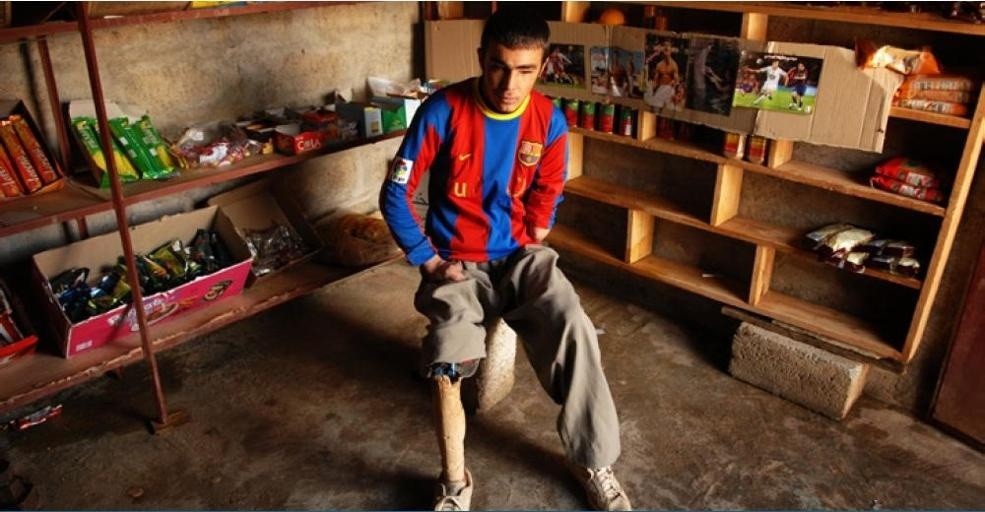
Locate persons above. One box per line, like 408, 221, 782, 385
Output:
536, 34, 813, 111
380, 9, 637, 512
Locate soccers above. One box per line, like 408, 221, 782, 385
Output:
805, 104, 812, 113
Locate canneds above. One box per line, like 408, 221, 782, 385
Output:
552, 99, 634, 137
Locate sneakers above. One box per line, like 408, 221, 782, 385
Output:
434, 465, 474, 511
572, 463, 632, 511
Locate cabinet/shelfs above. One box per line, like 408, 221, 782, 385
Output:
534, 0, 984, 377
0, 2, 453, 410
